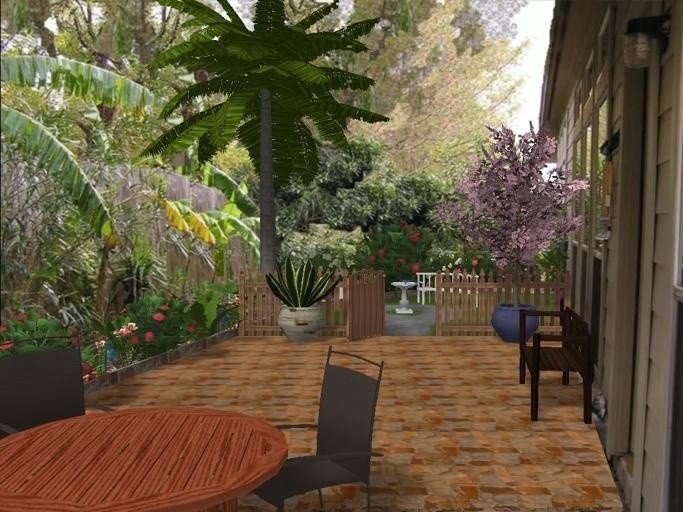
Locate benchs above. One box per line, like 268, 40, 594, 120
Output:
415, 271, 481, 308
518, 307, 597, 426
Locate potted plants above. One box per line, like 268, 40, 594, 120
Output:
265, 255, 343, 345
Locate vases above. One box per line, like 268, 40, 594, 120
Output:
492, 299, 539, 344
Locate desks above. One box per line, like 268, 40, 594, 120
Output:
1, 407, 287, 511
391, 282, 419, 314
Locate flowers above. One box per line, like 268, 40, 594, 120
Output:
439, 120, 593, 303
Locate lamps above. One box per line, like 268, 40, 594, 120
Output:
621, 13, 672, 69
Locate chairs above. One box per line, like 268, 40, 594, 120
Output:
254, 344, 385, 512
0, 346, 85, 438
